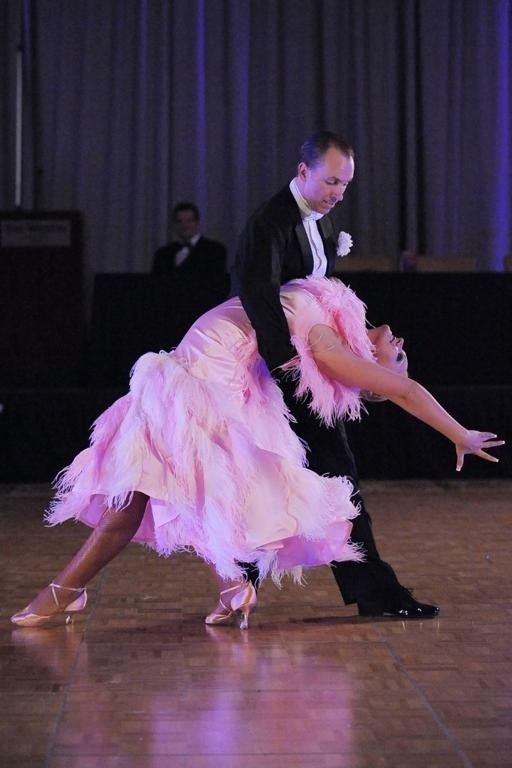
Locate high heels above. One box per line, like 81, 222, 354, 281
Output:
205, 581, 256, 629
10, 582, 87, 627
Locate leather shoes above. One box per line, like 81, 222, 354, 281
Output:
358, 585, 439, 619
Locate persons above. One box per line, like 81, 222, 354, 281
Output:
9, 272, 507, 632
398, 244, 426, 274
234, 132, 439, 625
145, 202, 228, 275
